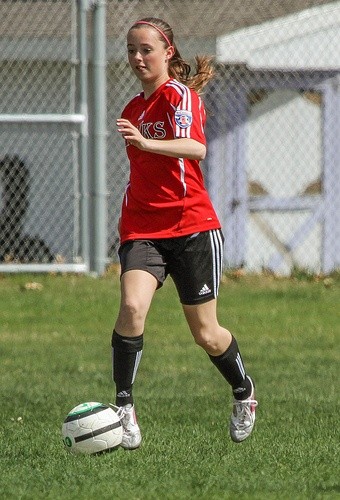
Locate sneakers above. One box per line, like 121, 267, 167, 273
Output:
230, 375, 258, 443
108, 402, 142, 449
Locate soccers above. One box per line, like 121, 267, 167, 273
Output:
61, 401, 124, 456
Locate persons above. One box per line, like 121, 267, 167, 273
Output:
112, 18, 259, 448
